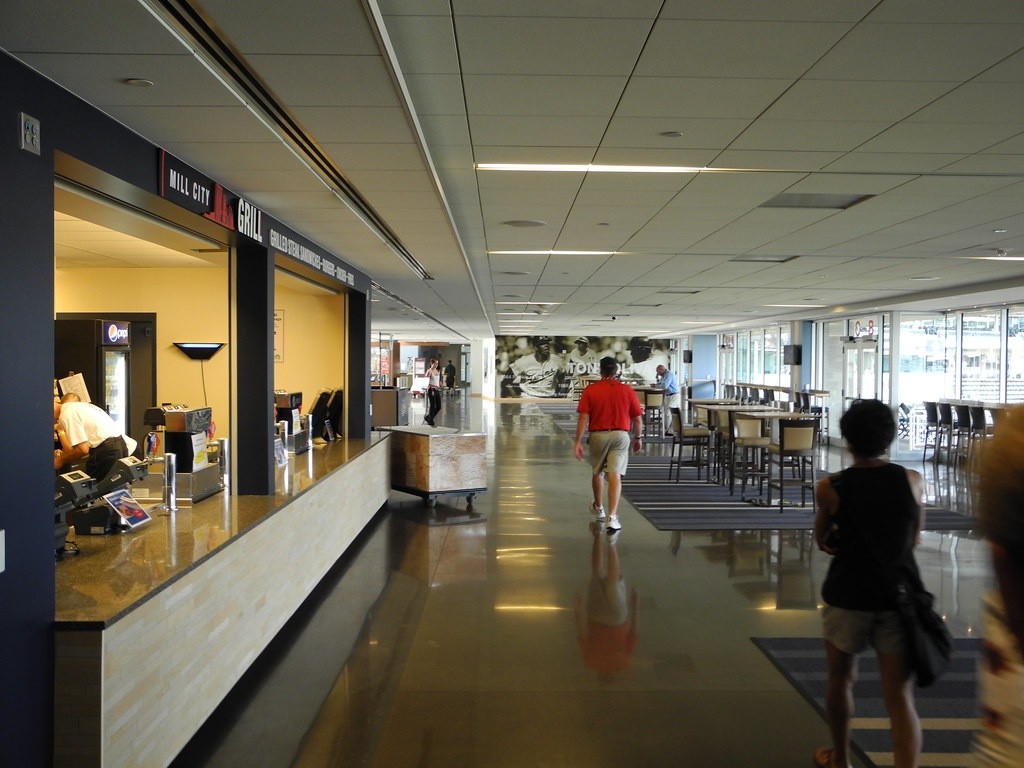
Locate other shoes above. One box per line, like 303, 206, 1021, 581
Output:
813, 745, 852, 768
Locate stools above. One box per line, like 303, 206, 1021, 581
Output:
569, 377, 830, 514
954, 404, 973, 474
922, 401, 941, 467
936, 402, 958, 468
969, 406, 994, 452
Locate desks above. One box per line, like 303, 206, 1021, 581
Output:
684, 398, 751, 469
694, 404, 784, 484
721, 382, 830, 444
934, 398, 1010, 421
632, 386, 665, 438
736, 412, 823, 507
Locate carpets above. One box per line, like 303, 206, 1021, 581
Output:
584, 455, 989, 531
552, 419, 715, 444
749, 636, 986, 768
536, 402, 581, 414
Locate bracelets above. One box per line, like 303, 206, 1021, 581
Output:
634, 437, 642, 439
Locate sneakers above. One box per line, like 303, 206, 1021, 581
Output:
605, 516, 621, 529
588, 498, 605, 518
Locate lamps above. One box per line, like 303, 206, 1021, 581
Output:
862, 335, 877, 342
172, 342, 226, 360
839, 336, 855, 342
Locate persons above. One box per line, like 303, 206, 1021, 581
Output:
649, 365, 679, 437
973, 405, 1024, 768
568, 337, 596, 398
425, 358, 444, 425
444, 360, 455, 389
813, 399, 924, 768
574, 357, 643, 530
621, 337, 669, 387
501, 336, 566, 398
54, 392, 129, 485
573, 523, 640, 682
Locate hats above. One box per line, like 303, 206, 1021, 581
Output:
626, 337, 652, 350
532, 336, 552, 343
573, 336, 589, 344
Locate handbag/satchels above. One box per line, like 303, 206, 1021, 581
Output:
901, 587, 955, 686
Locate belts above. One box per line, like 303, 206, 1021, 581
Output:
600, 428, 621, 431
667, 393, 674, 396
103, 435, 123, 445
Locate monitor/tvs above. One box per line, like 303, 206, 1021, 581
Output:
783, 345, 800, 365
684, 350, 692, 364
